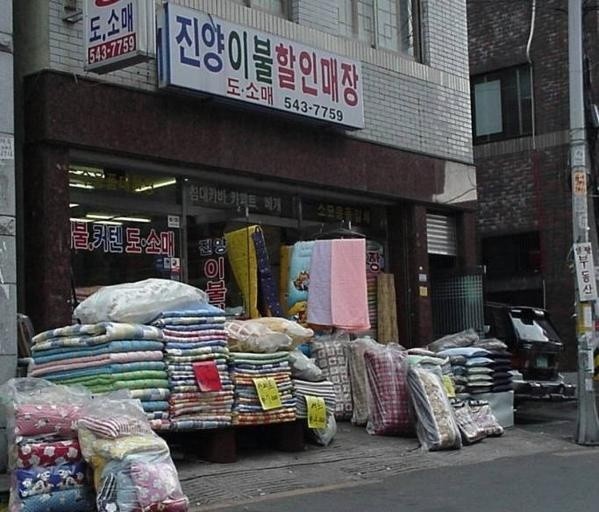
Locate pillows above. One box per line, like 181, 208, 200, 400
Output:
224, 317, 514, 454
5, 395, 189, 512
73, 278, 207, 328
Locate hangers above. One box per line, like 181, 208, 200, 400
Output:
307, 222, 344, 241
314, 219, 367, 241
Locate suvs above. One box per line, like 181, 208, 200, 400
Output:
481, 298, 578, 408
